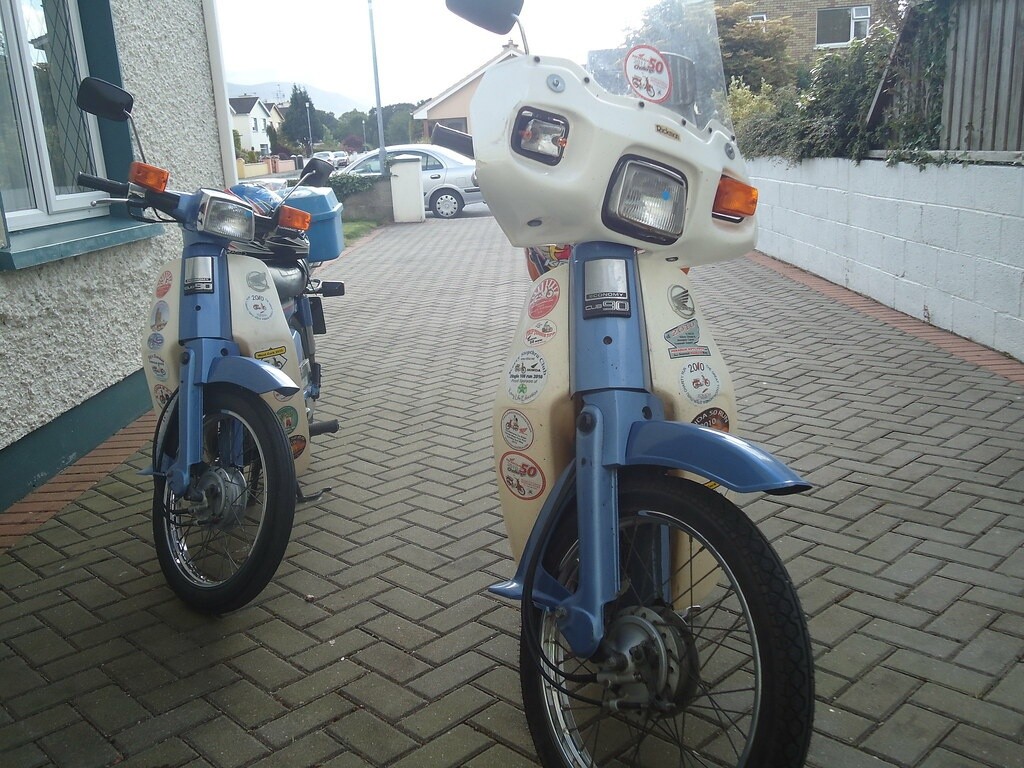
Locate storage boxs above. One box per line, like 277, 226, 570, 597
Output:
269, 187, 345, 261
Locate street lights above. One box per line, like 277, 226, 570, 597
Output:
363, 120, 367, 152
306, 102, 313, 153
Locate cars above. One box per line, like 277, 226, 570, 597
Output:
313, 151, 338, 171
333, 152, 350, 166
335, 142, 481, 218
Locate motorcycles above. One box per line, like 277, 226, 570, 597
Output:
72, 76, 346, 610
451, 1, 816, 768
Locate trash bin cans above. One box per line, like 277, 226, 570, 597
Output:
389, 154, 425, 223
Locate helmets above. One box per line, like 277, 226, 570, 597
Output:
225, 184, 285, 223
